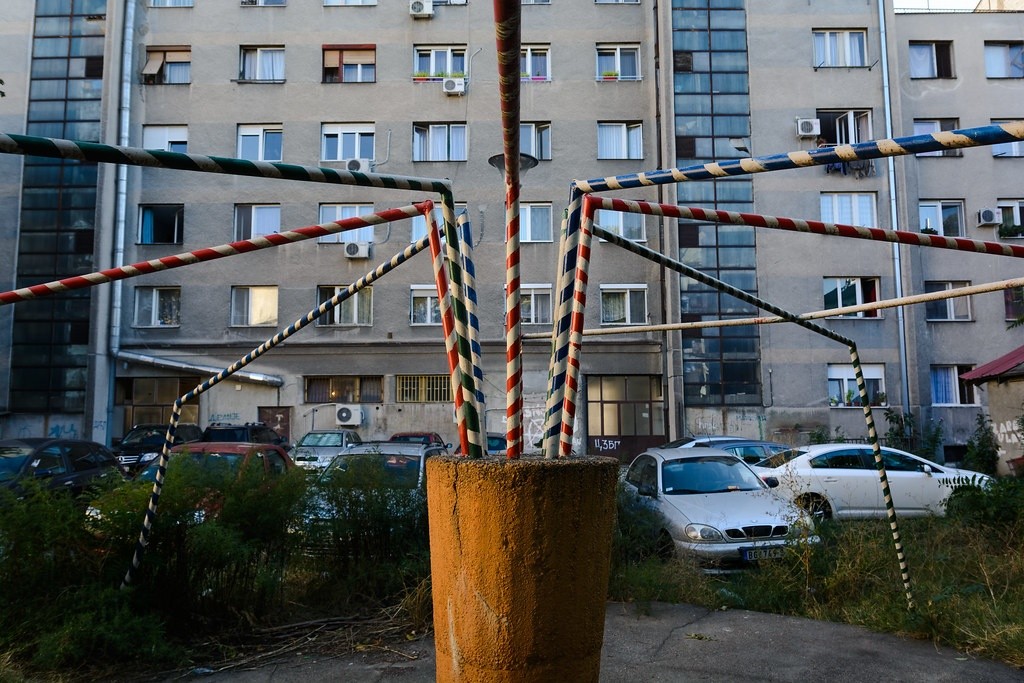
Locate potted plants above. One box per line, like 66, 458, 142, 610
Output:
829, 389, 887, 407
997, 223, 1024, 237
602, 72, 619, 82
520, 71, 546, 81
147, 76, 154, 84
414, 72, 464, 82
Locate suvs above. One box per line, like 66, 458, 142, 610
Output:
111, 423, 203, 476
201, 421, 295, 463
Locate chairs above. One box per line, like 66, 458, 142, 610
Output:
694, 465, 725, 493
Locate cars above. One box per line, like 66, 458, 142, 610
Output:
383, 432, 452, 468
617, 448, 820, 575
681, 439, 796, 489
453, 431, 508, 458
280, 428, 362, 483
74, 441, 306, 563
0, 437, 128, 503
641, 434, 790, 473
737, 443, 1003, 524
280, 441, 451, 565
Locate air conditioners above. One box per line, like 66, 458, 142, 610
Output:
335, 403, 361, 425
409, 0, 432, 16
442, 77, 464, 94
346, 158, 371, 173
796, 118, 820, 137
344, 242, 368, 257
441, 241, 462, 257
976, 208, 1002, 227
